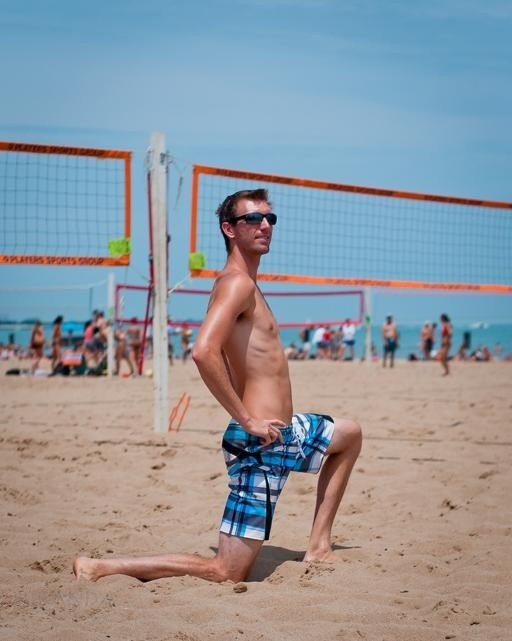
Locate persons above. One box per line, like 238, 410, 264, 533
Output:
72, 185, 361, 586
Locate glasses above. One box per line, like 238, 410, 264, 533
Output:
231, 211, 277, 226
